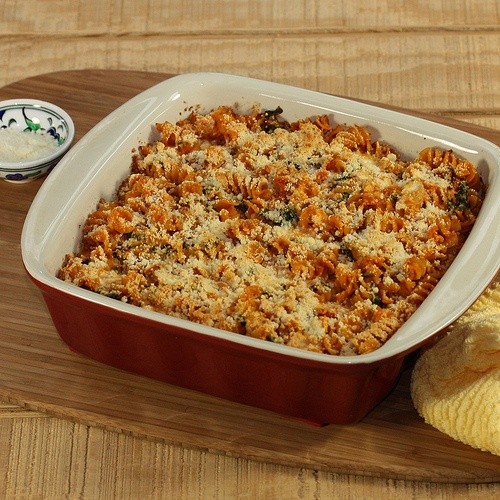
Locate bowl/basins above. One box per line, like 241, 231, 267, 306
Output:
18, 73, 500, 426
0, 98, 75, 186
